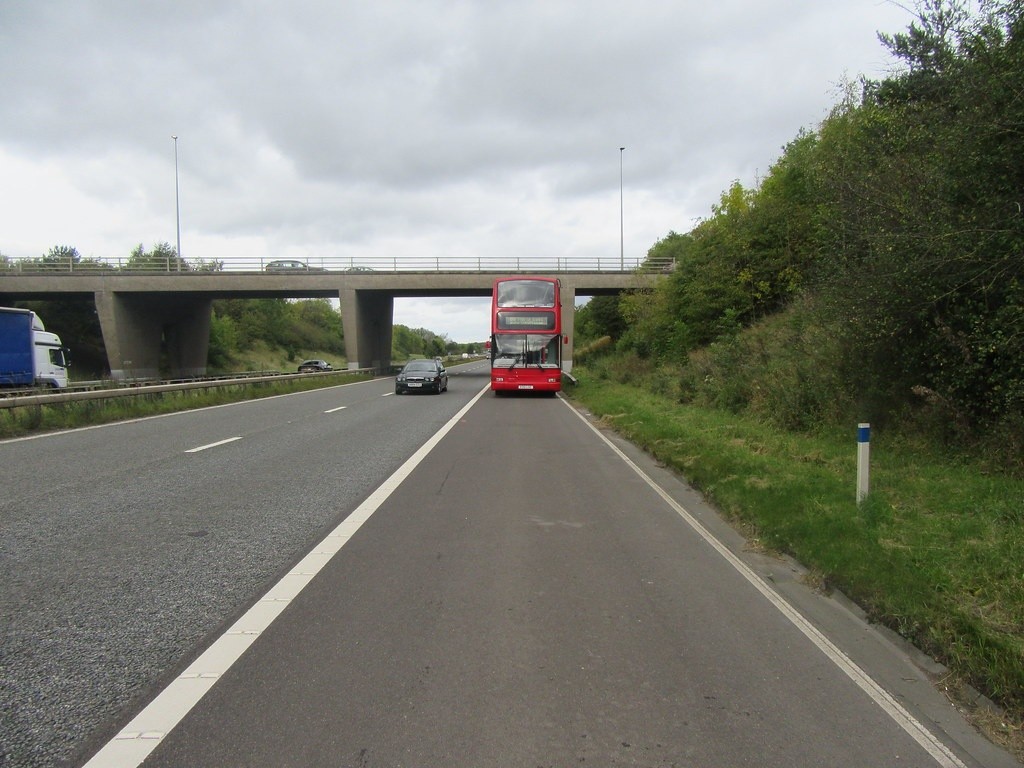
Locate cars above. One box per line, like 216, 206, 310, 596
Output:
343, 266, 377, 271
298, 360, 333, 373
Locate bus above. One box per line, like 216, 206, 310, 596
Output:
486, 276, 569, 397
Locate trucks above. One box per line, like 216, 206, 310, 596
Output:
0, 307, 72, 398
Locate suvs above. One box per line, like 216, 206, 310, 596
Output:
266, 260, 328, 271
395, 358, 448, 395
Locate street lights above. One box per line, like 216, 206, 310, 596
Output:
171, 135, 181, 271
620, 148, 625, 271
